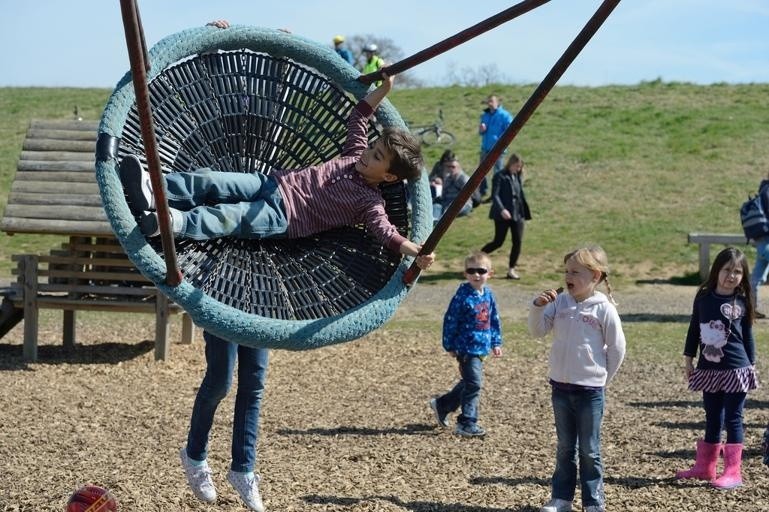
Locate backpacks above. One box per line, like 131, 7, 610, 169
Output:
739, 185, 768, 239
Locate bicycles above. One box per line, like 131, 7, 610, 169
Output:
405, 109, 454, 150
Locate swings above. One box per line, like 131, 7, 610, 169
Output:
96, 0, 618, 350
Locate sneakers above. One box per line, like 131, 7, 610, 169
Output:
430, 397, 448, 427
541, 499, 606, 511
121, 153, 155, 209
180, 448, 217, 502
753, 309, 765, 320
225, 469, 264, 512
139, 210, 162, 239
507, 270, 520, 280
456, 423, 486, 437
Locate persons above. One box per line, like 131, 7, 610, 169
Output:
362, 44, 385, 88
429, 251, 503, 436
529, 245, 627, 512
749, 179, 769, 318
180, 21, 291, 512
428, 95, 514, 225
120, 63, 435, 270
481, 153, 532, 279
329, 37, 354, 104
676, 248, 759, 488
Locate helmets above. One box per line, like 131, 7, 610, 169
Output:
333, 35, 345, 44
362, 44, 378, 53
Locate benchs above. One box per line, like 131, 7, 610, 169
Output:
686, 232, 755, 282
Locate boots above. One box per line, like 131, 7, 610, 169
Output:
677, 439, 722, 479
713, 443, 743, 487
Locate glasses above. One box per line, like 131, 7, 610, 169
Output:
466, 267, 488, 276
